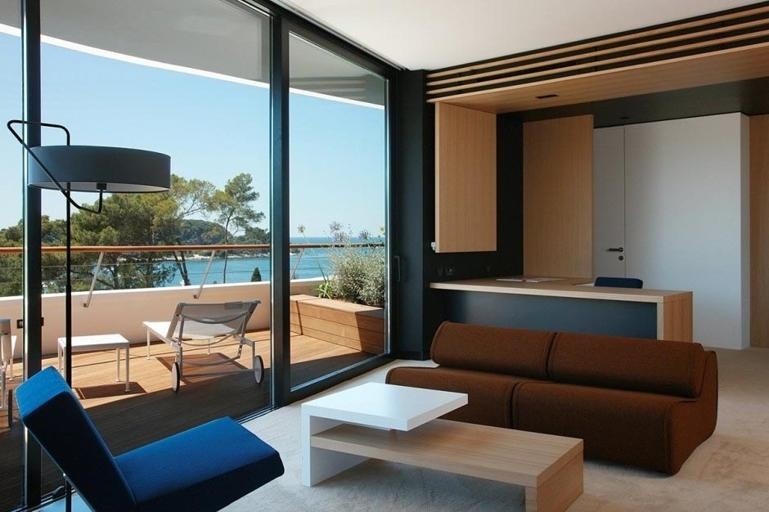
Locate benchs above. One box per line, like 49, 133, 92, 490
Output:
289, 294, 383, 356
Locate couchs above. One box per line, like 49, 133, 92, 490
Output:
382, 319, 720, 476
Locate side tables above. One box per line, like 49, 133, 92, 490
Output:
58, 334, 130, 392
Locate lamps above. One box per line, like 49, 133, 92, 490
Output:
3, 119, 171, 511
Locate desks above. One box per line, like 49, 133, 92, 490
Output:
428, 275, 693, 342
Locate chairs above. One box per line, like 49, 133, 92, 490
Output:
15, 365, 285, 511
142, 300, 265, 393
595, 277, 643, 288
0, 319, 17, 409
